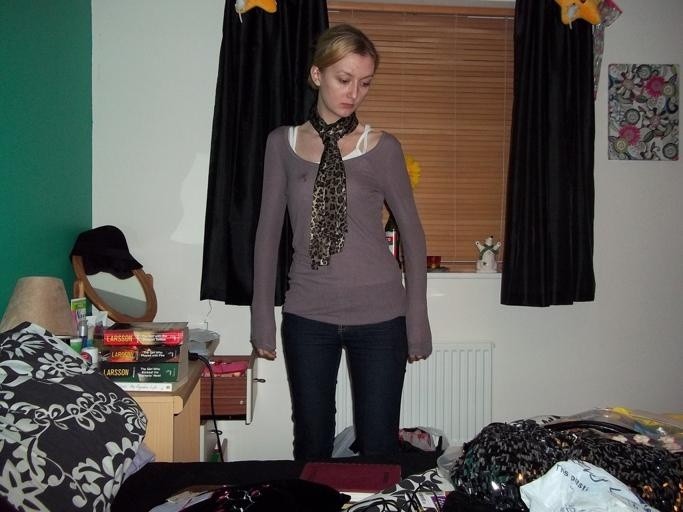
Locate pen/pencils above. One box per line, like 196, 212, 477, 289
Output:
175, 498, 193, 512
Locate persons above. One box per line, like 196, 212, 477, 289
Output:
248, 24, 432, 456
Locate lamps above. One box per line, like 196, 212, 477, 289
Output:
0, 272, 81, 345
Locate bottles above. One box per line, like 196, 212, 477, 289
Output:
386, 229, 399, 264
69, 320, 92, 365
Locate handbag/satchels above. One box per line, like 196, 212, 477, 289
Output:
182, 476, 352, 512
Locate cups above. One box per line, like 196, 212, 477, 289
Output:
426, 255, 441, 268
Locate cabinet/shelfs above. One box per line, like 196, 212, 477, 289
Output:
102, 333, 265, 464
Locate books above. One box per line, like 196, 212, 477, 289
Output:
300, 461, 406, 493
101, 319, 189, 385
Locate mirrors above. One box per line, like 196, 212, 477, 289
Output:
68, 242, 160, 327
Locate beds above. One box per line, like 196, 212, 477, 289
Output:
2, 293, 680, 511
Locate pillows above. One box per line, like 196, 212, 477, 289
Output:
1, 316, 147, 512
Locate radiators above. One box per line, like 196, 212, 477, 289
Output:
326, 338, 496, 455
